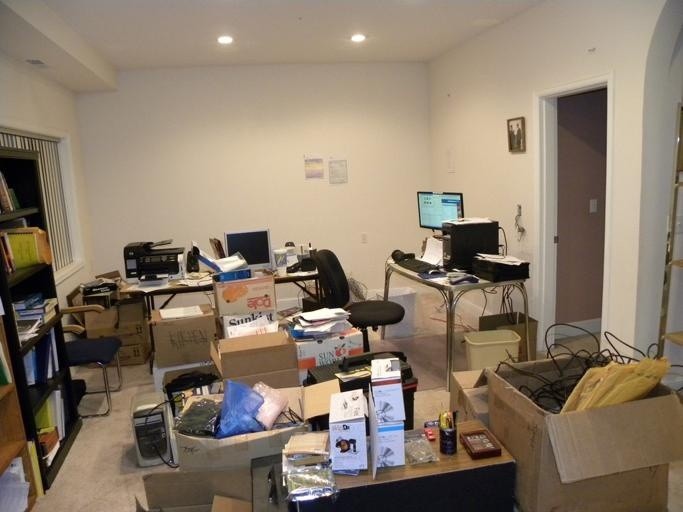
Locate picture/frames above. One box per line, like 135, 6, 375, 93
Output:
460, 430, 501, 459
506, 115, 526, 155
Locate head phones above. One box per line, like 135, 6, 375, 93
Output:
391, 249, 415, 262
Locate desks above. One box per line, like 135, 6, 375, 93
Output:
381, 253, 532, 392
295, 418, 518, 512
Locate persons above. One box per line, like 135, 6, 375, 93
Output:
515, 123, 521, 148
339, 440, 350, 453
510, 124, 515, 149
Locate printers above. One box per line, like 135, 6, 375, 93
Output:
123, 239, 186, 284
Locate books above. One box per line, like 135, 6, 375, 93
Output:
284, 432, 332, 465
291, 306, 355, 340
177, 271, 212, 287
0, 170, 68, 510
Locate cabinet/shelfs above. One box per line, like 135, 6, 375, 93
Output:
0, 147, 84, 512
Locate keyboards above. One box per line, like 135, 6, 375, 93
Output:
398, 258, 440, 274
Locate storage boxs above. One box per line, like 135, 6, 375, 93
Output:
474, 355, 683, 512
479, 311, 539, 360
133, 464, 288, 512
150, 305, 217, 367
170, 377, 341, 471
215, 332, 299, 388
85, 304, 149, 369
449, 370, 489, 430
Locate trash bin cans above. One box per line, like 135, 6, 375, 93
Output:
463, 330, 522, 371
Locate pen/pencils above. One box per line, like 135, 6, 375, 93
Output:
437, 410, 459, 429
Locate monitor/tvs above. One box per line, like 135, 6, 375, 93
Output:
224, 228, 271, 269
417, 190, 464, 231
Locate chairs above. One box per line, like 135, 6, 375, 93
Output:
315, 250, 405, 353
61, 304, 121, 418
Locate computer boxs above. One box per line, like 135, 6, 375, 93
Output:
442, 216, 499, 272
130, 389, 169, 468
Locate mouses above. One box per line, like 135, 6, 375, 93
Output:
428, 269, 441, 274
